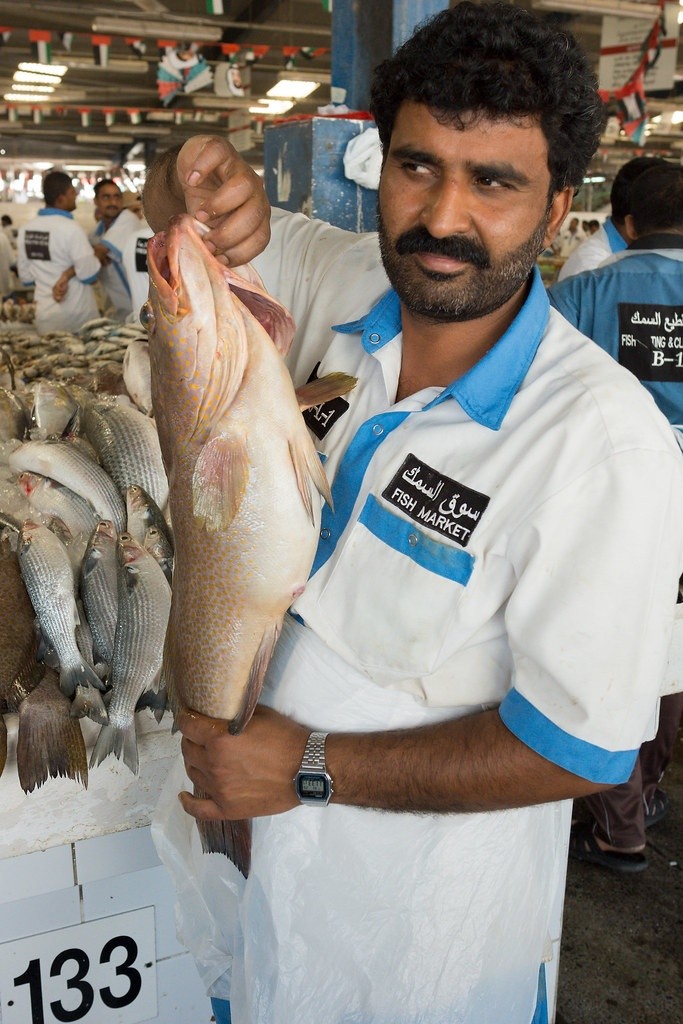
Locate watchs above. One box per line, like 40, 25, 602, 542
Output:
292, 732, 334, 807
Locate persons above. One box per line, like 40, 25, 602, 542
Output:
141, 1, 683, 1024
0, 215, 19, 297
52, 178, 143, 321
15, 171, 102, 335
546, 156, 683, 870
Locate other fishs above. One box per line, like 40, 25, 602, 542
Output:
1, 317, 174, 796
120, 213, 356, 880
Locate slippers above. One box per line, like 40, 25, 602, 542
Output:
569, 822, 649, 873
642, 787, 671, 830
